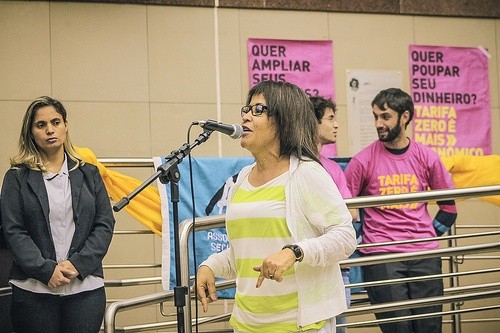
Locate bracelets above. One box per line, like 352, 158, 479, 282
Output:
282, 244, 303, 262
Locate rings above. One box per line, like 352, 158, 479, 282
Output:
269, 275, 274, 279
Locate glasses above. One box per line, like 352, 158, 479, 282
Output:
241, 104, 267, 116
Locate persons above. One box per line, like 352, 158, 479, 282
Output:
191, 82, 357, 333
306, 97, 359, 333
341, 86, 458, 333
1, 96, 115, 333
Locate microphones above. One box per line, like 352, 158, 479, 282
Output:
193, 119, 243, 139
